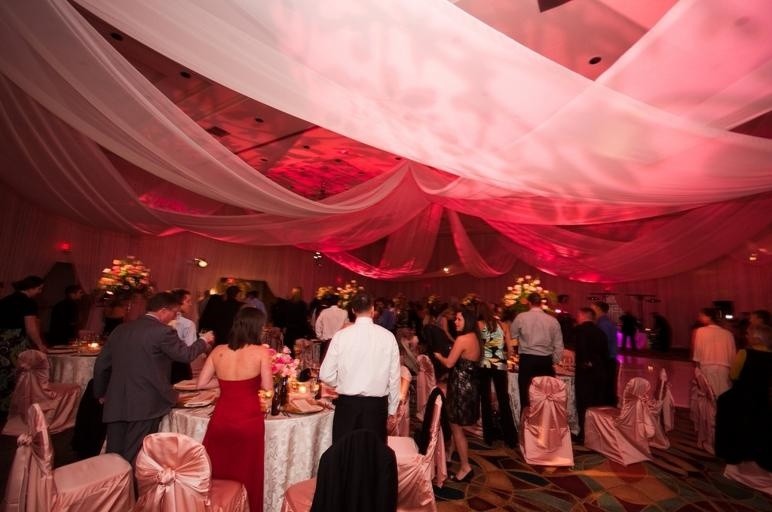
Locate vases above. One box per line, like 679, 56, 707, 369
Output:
272, 379, 287, 415
102, 304, 123, 335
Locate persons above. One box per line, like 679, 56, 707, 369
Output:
50, 283, 86, 345
201, 283, 398, 352
618, 309, 640, 350
690, 293, 772, 462
431, 308, 483, 482
649, 310, 672, 349
0, 275, 47, 381
169, 289, 201, 382
91, 292, 215, 457
197, 305, 274, 512
422, 285, 622, 444
319, 292, 399, 445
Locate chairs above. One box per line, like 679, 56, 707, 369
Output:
554, 351, 575, 374
386, 386, 443, 512
1, 350, 81, 436
386, 365, 412, 437
265, 327, 284, 353
401, 337, 417, 373
415, 355, 447, 422
585, 366, 772, 495
0, 402, 136, 512
295, 338, 320, 365
518, 376, 574, 467
280, 430, 397, 512
134, 433, 250, 512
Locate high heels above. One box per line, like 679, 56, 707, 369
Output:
450, 468, 475, 484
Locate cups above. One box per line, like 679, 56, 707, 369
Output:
201, 328, 210, 333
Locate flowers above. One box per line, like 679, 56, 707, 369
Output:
318, 280, 363, 306
502, 276, 560, 313
96, 255, 155, 304
263, 344, 296, 378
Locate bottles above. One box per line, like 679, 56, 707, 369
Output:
72, 331, 96, 354
272, 375, 289, 416
316, 373, 322, 399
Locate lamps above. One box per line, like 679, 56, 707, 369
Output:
193, 258, 208, 268
